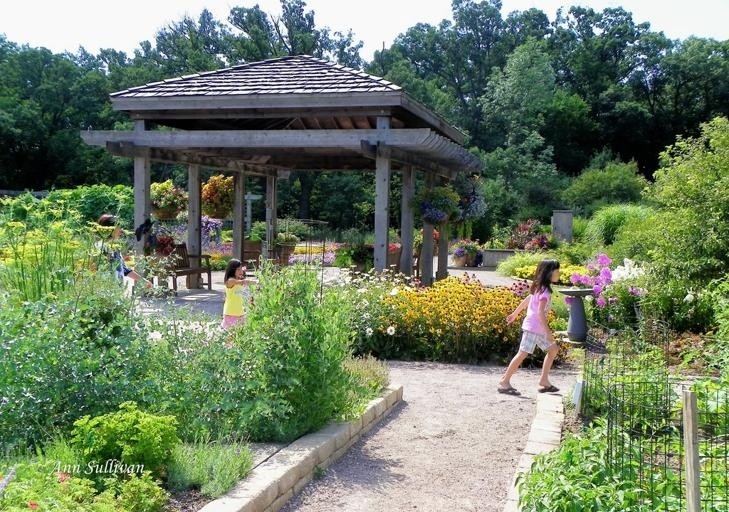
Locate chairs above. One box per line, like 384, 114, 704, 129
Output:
166, 243, 213, 297
243, 240, 262, 269
411, 243, 423, 278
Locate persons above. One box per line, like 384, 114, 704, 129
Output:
94, 214, 153, 290
221, 259, 258, 345
498, 258, 560, 395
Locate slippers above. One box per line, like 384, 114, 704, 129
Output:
498, 387, 521, 396
538, 385, 559, 393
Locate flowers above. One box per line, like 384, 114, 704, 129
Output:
199, 174, 236, 208
455, 244, 480, 256
387, 243, 401, 254
136, 219, 178, 259
150, 186, 188, 210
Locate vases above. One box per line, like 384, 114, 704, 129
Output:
208, 208, 226, 218
455, 256, 476, 267
155, 211, 179, 218
